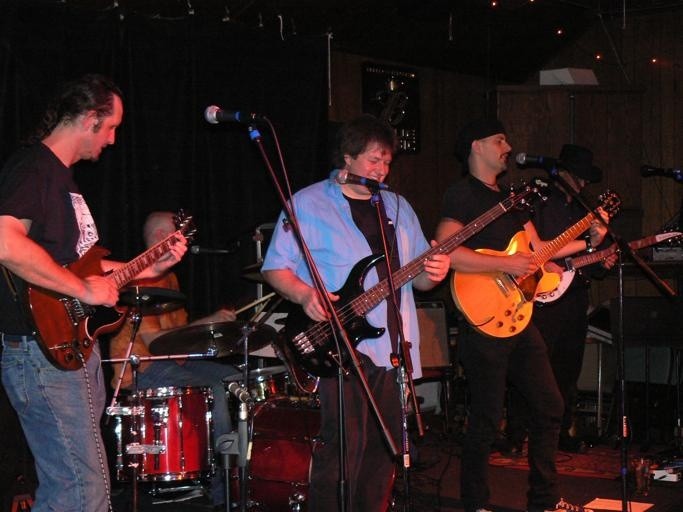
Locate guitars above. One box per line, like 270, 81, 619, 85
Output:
532, 227, 682, 303
270, 174, 555, 394
21, 206, 201, 372
449, 189, 623, 342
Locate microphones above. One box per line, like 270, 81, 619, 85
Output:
191, 245, 229, 255
227, 381, 255, 407
204, 105, 264, 124
640, 164, 681, 178
516, 152, 563, 170
337, 169, 389, 190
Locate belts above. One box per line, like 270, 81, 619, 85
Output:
1, 333, 33, 343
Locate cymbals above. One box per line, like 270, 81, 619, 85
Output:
113, 285, 191, 316
222, 366, 287, 382
148, 319, 278, 360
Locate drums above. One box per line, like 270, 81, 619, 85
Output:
231, 395, 397, 511
117, 386, 218, 483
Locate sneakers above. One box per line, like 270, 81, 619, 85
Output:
544, 497, 593, 512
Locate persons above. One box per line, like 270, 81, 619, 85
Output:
260, 112, 452, 512
497, 143, 618, 457
0, 74, 189, 512
111, 211, 240, 511
436, 112, 607, 512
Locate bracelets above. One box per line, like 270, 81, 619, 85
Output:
585, 234, 594, 253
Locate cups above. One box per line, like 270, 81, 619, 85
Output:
631, 458, 653, 498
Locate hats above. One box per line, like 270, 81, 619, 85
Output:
555, 143, 603, 184
